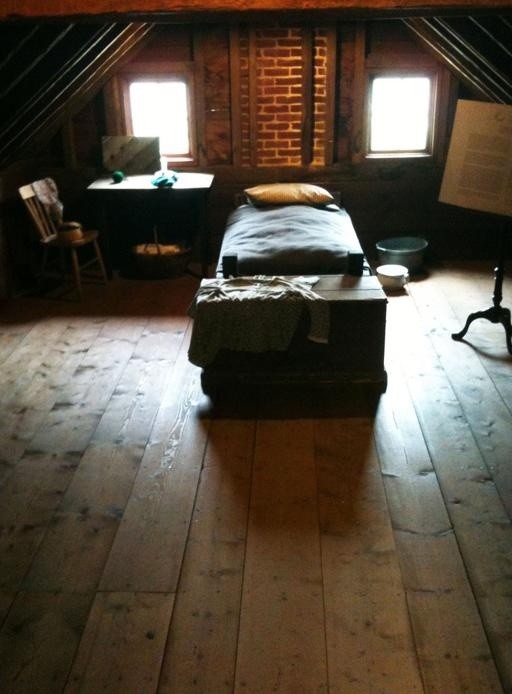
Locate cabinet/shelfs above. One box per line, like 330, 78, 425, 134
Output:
197, 277, 389, 414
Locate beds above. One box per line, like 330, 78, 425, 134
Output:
212, 191, 373, 277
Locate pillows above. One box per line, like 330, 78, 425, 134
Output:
244, 183, 334, 208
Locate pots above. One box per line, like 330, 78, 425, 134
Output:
374, 233, 430, 277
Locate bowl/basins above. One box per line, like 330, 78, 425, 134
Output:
376, 263, 410, 292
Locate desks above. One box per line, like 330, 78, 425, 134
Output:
87, 172, 215, 281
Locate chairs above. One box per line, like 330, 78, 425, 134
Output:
18, 178, 108, 299
